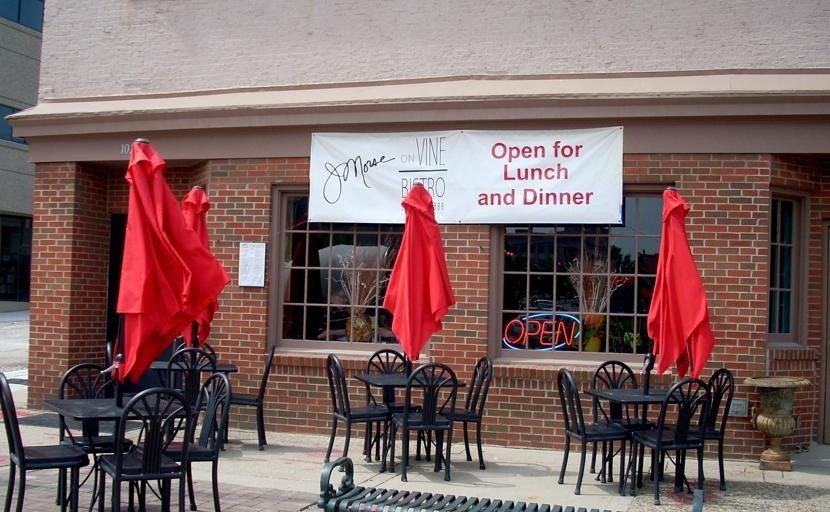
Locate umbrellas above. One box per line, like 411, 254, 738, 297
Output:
180, 184, 221, 367
115, 137, 230, 408
383, 184, 456, 381
645, 188, 714, 395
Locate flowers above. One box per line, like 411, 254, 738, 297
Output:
624, 332, 641, 350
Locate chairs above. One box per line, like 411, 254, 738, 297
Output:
55, 363, 133, 511
0, 372, 90, 512
175, 338, 217, 364
426, 356, 492, 470
97, 387, 192, 512
630, 378, 712, 505
363, 348, 422, 461
590, 360, 655, 482
557, 368, 629, 495
106, 341, 112, 367
136, 373, 232, 512
389, 362, 458, 482
324, 354, 390, 471
167, 348, 216, 449
221, 345, 275, 451
659, 368, 735, 491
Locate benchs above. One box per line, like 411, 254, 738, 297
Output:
318, 458, 705, 512
